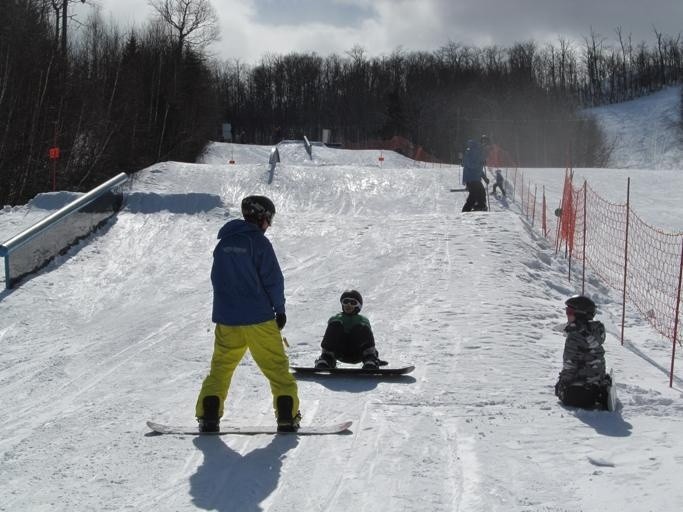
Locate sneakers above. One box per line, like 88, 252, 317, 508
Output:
197, 415, 220, 431
277, 413, 302, 432
316, 356, 329, 367
362, 359, 378, 370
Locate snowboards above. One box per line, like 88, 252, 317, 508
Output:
608, 369, 618, 411
451, 188, 489, 192
147, 420, 352, 433
290, 365, 414, 376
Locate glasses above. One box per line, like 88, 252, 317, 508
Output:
566, 306, 576, 314
341, 298, 360, 306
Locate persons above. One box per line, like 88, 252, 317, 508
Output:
553, 296, 614, 412
314, 289, 388, 372
194, 195, 303, 432
460, 133, 491, 212
489, 169, 506, 196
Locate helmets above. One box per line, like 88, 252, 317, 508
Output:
241, 194, 275, 222
564, 296, 596, 320
340, 289, 362, 310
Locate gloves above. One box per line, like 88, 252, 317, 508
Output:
276, 313, 286, 330
